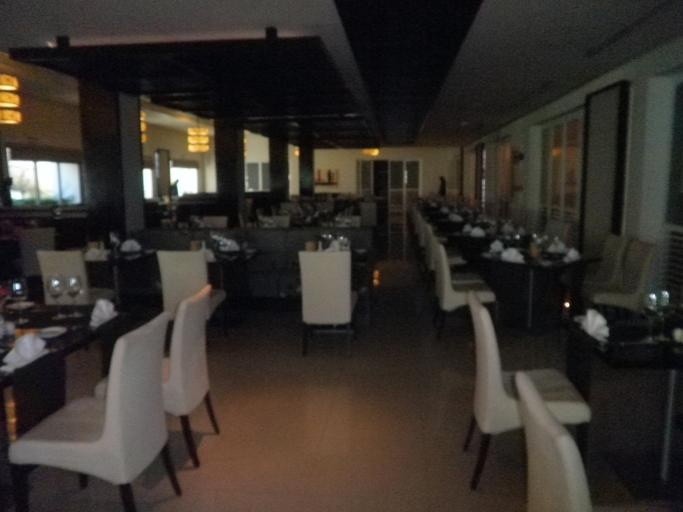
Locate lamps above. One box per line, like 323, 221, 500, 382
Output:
0, 73, 22, 126
185, 117, 211, 153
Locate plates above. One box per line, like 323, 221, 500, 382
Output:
37, 326, 65, 339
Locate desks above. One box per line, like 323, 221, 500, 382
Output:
0, 300, 125, 511
543, 306, 683, 485
127, 227, 368, 310
481, 250, 574, 333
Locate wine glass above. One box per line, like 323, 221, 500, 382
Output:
644, 290, 675, 343
47, 275, 84, 320
8, 279, 31, 324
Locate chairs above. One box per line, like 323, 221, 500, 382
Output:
575, 230, 656, 307
9, 308, 183, 511
154, 247, 226, 326
408, 202, 500, 341
33, 248, 92, 304
298, 249, 359, 357
457, 287, 596, 494
512, 369, 596, 510
93, 283, 221, 470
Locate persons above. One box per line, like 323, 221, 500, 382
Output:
438, 176, 446, 196
